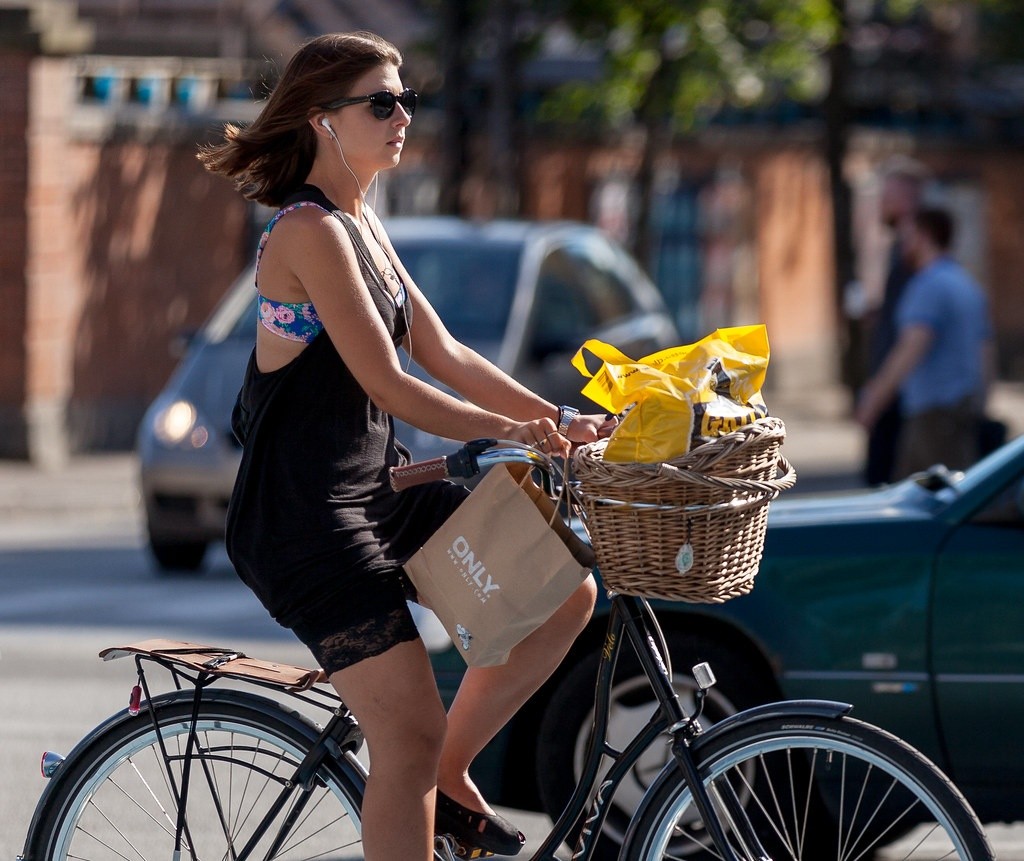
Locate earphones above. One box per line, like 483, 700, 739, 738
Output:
321, 118, 336, 138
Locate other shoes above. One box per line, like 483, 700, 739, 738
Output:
435, 788, 526, 855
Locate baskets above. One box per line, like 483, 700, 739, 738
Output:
571, 417, 797, 604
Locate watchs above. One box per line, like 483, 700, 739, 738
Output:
558, 405, 580, 436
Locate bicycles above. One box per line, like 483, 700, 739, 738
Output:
19, 414, 997, 861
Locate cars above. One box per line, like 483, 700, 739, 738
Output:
563, 436, 1024, 823
137, 215, 684, 576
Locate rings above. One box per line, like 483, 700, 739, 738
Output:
546, 432, 557, 437
540, 439, 547, 445
531, 442, 538, 447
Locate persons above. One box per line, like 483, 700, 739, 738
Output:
853, 199, 993, 478
198, 33, 617, 859
864, 169, 925, 487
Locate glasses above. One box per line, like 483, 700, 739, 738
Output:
322, 87, 417, 120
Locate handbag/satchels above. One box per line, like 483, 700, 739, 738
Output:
570, 324, 770, 461
402, 447, 595, 667
